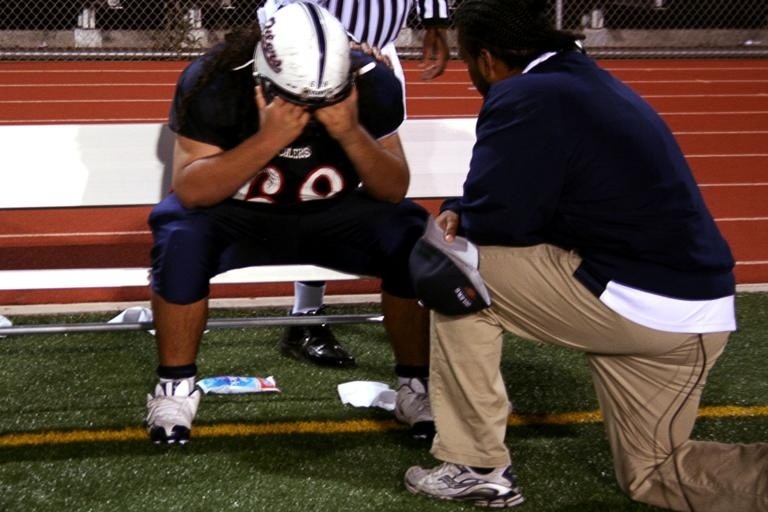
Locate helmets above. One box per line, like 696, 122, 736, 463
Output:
252, 2, 352, 108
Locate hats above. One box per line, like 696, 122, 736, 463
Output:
449, 0, 586, 51
409, 214, 492, 316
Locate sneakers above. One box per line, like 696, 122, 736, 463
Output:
145, 378, 201, 446
395, 376, 437, 435
280, 304, 355, 368
403, 462, 525, 508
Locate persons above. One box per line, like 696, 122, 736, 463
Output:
404, 1, 768, 512
146, 2, 437, 446
271, 0, 452, 367
